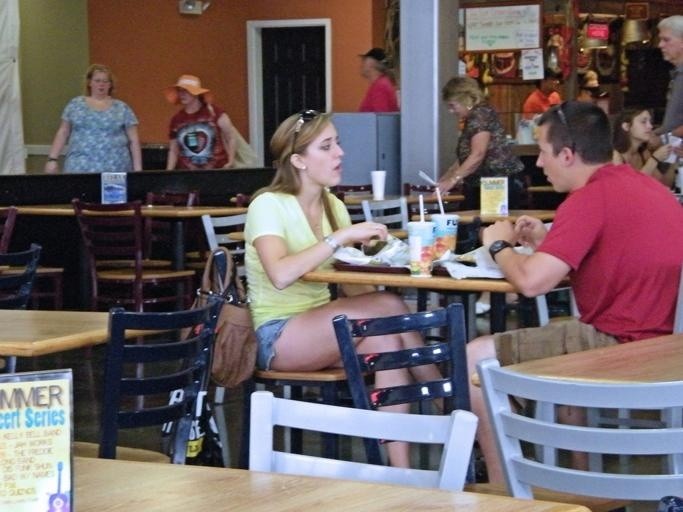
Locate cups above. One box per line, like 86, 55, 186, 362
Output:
406, 221, 433, 277
430, 214, 459, 259
370, 171, 386, 201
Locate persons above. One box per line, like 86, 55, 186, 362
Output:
522, 15, 682, 191
159, 74, 237, 171
44, 64, 142, 174
242, 109, 446, 469
466, 99, 683, 487
427, 77, 525, 210
355, 48, 400, 112
384, 71, 400, 109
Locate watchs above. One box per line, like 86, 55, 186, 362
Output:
488, 239, 513, 262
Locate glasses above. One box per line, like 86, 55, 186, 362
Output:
538, 100, 575, 153
291, 110, 320, 154
92, 78, 112, 84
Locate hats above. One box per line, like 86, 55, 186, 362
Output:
358, 48, 386, 64
163, 75, 213, 105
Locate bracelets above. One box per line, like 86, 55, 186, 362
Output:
323, 235, 339, 253
451, 171, 463, 186
47, 158, 56, 161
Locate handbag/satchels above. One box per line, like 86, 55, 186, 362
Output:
162, 388, 225, 466
181, 290, 257, 389
222, 124, 258, 167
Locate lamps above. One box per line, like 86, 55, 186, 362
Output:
178, 0, 211, 16
